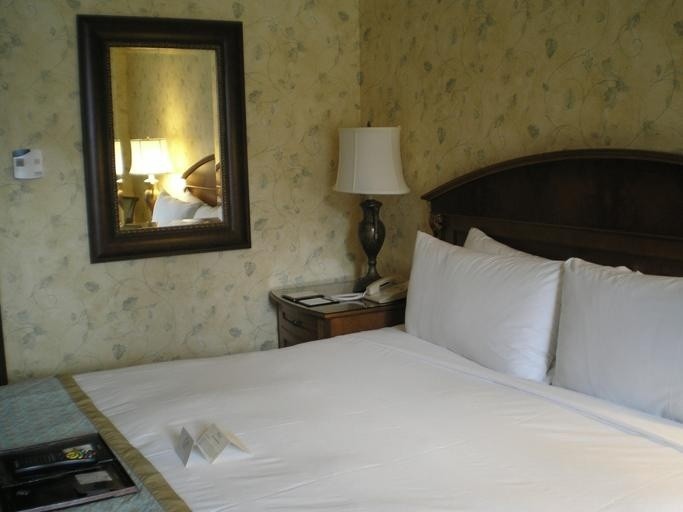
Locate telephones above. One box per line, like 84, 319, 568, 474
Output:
362, 276, 406, 305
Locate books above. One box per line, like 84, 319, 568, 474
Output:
0, 433, 138, 512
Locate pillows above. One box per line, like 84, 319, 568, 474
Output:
149, 189, 219, 221
462, 227, 553, 262
549, 253, 682, 423
407, 230, 563, 387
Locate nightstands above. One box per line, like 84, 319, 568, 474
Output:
268, 276, 406, 350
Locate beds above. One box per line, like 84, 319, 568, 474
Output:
0, 149, 683, 511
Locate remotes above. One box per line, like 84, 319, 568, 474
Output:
8, 449, 98, 477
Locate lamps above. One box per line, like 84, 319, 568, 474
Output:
332, 121, 409, 293
125, 136, 170, 215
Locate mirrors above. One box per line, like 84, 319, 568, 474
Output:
74, 14, 252, 264
